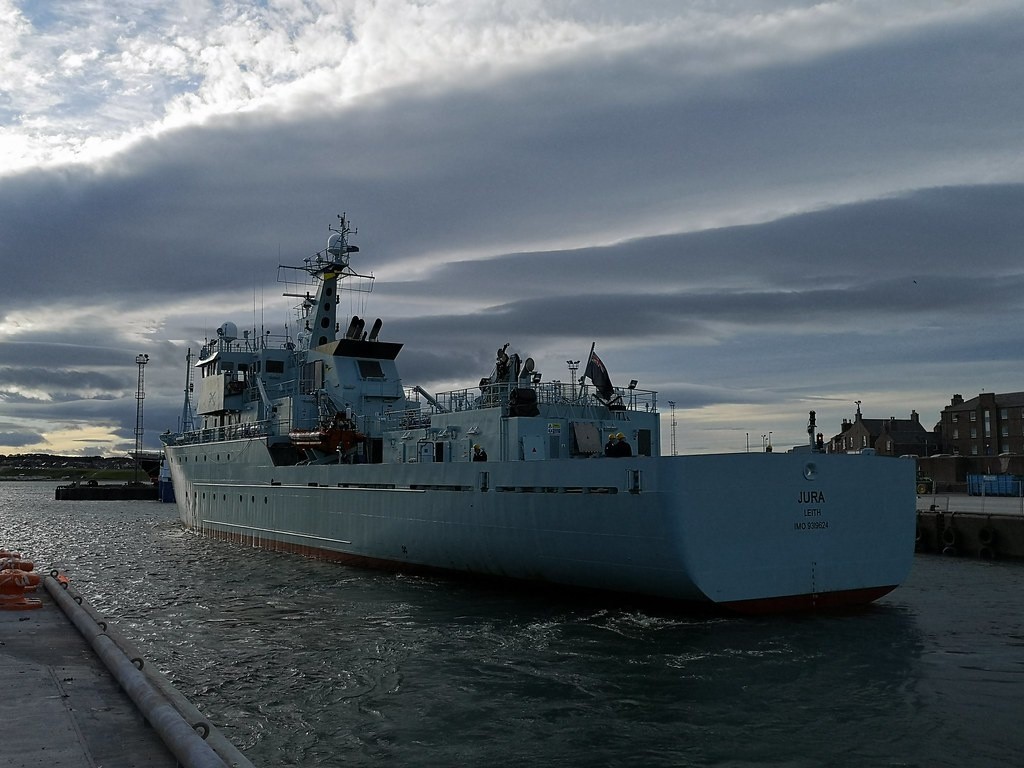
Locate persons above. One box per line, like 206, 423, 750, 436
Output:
765, 445, 773, 452
605, 432, 632, 457
472, 443, 487, 461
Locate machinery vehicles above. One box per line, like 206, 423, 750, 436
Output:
916, 470, 938, 496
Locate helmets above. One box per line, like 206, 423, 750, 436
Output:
608, 434, 616, 439
615, 432, 626, 439
473, 444, 481, 449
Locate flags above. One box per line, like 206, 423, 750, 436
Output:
585, 351, 616, 401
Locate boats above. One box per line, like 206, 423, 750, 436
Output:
159, 210, 918, 622
288, 427, 365, 460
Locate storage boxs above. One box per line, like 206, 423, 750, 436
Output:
966, 473, 1024, 497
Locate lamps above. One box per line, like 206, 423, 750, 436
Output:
468, 427, 480, 435
628, 379, 639, 390
533, 373, 542, 383
439, 429, 449, 438
401, 432, 414, 440
